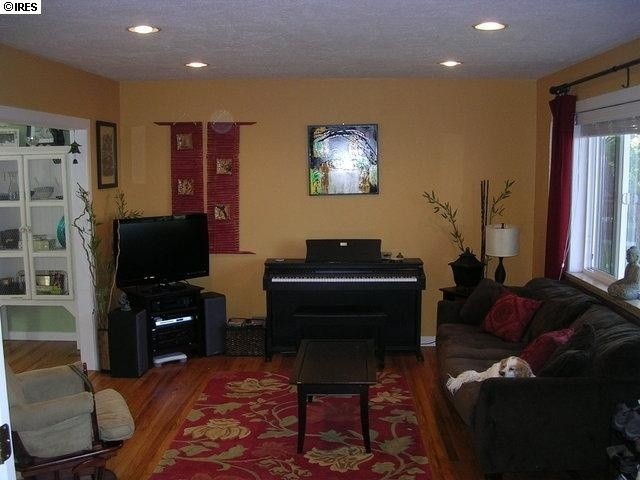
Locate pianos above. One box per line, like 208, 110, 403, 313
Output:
263, 238, 426, 363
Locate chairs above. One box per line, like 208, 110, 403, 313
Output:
4, 360, 136, 480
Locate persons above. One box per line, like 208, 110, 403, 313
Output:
607, 245, 640, 300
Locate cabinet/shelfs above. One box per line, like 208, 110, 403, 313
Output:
0, 145, 81, 349
127, 282, 205, 369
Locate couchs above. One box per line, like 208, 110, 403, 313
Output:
436, 275, 640, 480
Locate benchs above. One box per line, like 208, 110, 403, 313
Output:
291, 306, 389, 368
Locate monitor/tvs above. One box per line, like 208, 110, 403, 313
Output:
113, 211, 210, 297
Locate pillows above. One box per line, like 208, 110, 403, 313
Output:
455, 277, 595, 379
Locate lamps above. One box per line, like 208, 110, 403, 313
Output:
485, 224, 520, 288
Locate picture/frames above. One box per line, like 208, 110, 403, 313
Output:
96, 120, 118, 189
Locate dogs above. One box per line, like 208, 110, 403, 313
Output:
446, 355, 537, 397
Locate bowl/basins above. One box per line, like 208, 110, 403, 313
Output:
34, 186, 56, 200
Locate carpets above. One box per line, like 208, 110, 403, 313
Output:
148, 371, 433, 480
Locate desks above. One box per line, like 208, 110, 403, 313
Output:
439, 286, 518, 302
